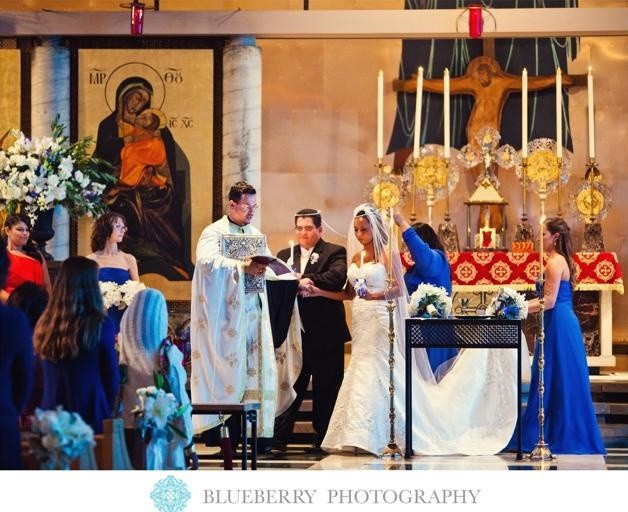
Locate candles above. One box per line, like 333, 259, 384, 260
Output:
444, 67, 449, 157
360, 250, 366, 274
388, 218, 392, 276
413, 66, 424, 157
538, 220, 544, 280
586, 66, 595, 157
555, 67, 563, 157
521, 67, 528, 157
376, 67, 385, 158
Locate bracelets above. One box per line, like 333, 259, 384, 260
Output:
382, 289, 393, 300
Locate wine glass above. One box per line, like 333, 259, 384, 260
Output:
458, 288, 472, 313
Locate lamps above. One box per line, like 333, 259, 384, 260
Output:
467, 2, 483, 41
129, 0, 146, 35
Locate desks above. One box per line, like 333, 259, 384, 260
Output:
403, 315, 524, 463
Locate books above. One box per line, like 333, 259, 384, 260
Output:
221, 232, 265, 293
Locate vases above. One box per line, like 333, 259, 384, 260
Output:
19, 203, 55, 261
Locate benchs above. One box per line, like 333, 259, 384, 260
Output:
189, 402, 262, 468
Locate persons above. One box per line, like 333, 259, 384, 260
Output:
113, 108, 171, 192
0, 206, 193, 469
90, 76, 191, 283
394, 56, 590, 143
297, 203, 527, 454
253, 206, 350, 457
187, 177, 316, 468
502, 209, 606, 458
385, 206, 460, 378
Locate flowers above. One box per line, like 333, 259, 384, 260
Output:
308, 252, 319, 265
19, 407, 96, 469
0, 114, 119, 230
130, 385, 183, 445
290, 240, 294, 260
97, 278, 146, 312
353, 276, 369, 298
484, 286, 530, 321
411, 282, 454, 317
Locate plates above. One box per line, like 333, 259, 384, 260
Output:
454, 313, 496, 319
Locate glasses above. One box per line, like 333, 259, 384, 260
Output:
240, 202, 259, 209
113, 223, 127, 232
